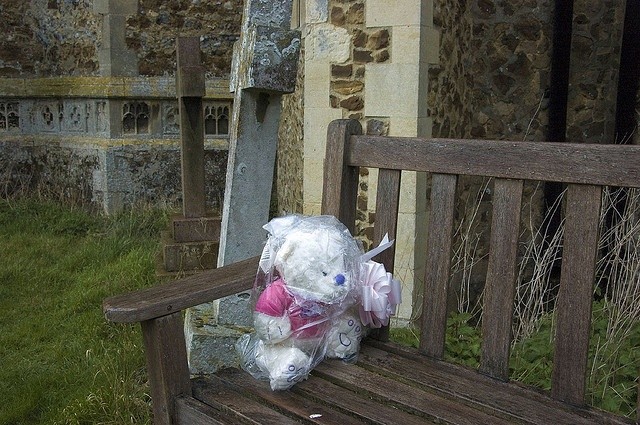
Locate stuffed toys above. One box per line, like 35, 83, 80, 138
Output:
255, 226, 360, 392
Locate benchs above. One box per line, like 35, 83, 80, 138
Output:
102, 120, 639, 424
